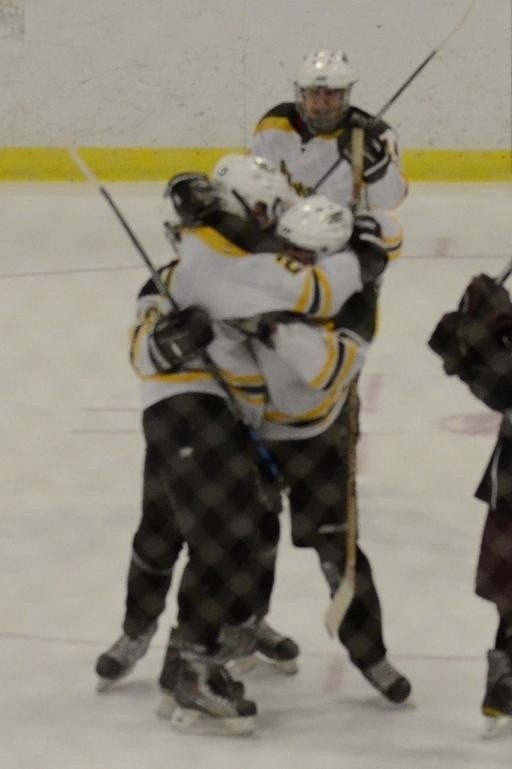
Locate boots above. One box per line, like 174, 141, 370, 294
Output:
483, 649, 512, 718
96, 619, 157, 679
360, 655, 410, 703
160, 615, 299, 717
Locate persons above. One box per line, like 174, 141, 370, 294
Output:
429, 271, 512, 722
96, 152, 411, 736
250, 48, 411, 215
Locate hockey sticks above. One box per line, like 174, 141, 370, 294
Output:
307, 1, 484, 197
59, 136, 294, 499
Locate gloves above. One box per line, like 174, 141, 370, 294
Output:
428, 275, 512, 411
167, 171, 221, 227
222, 311, 304, 350
347, 202, 388, 277
338, 112, 390, 184
148, 305, 214, 375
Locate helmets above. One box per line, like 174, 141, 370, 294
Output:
211, 154, 288, 232
291, 49, 359, 135
275, 195, 355, 264
